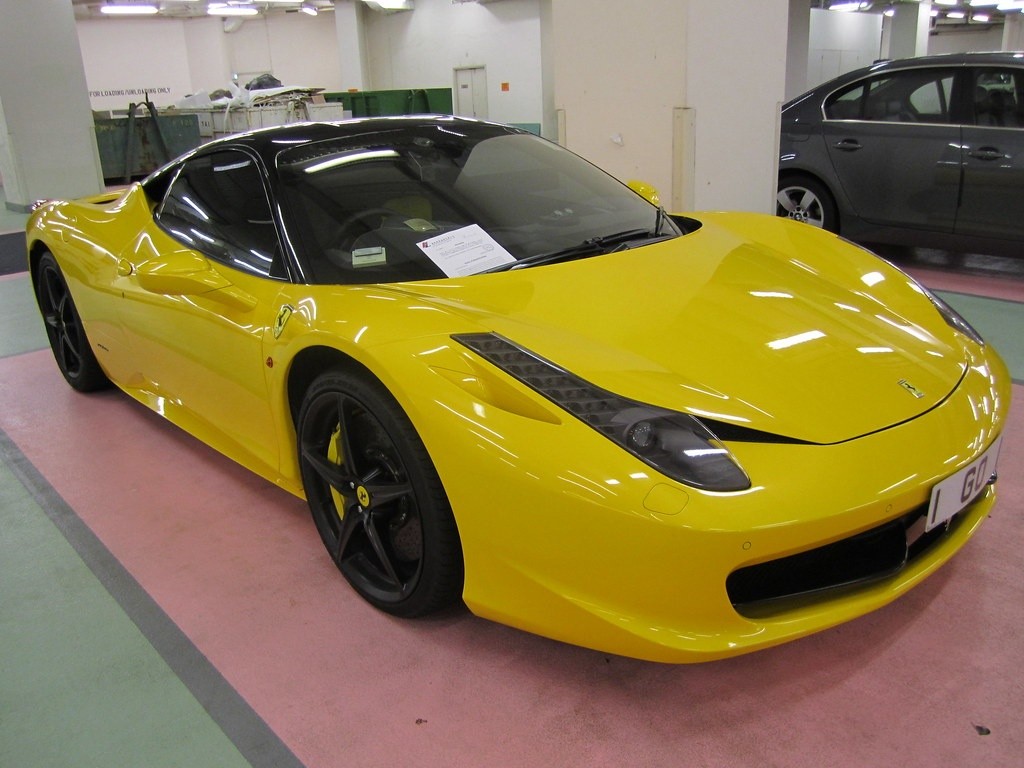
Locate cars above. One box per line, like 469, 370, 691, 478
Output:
777, 50, 1024, 260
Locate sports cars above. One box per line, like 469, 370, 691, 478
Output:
25, 113, 1007, 664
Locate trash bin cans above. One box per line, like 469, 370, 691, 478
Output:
95, 87, 453, 187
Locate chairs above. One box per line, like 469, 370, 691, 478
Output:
986, 89, 1017, 126
380, 196, 433, 224
975, 86, 992, 123
